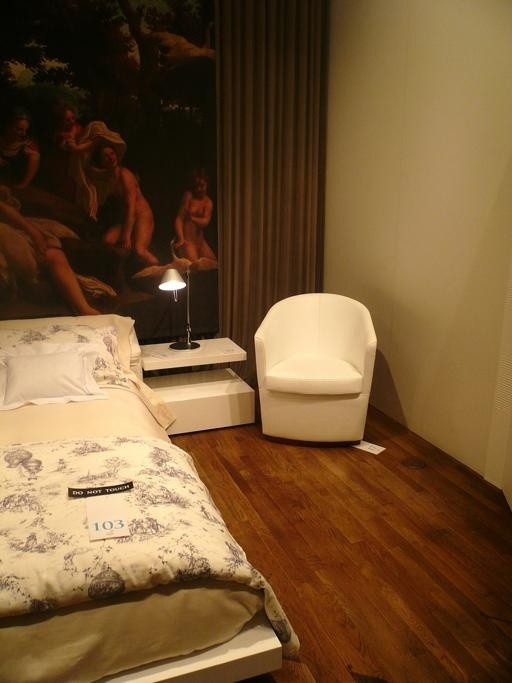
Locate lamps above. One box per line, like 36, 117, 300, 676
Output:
158, 268, 201, 351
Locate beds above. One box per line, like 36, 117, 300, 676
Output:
0, 314, 302, 683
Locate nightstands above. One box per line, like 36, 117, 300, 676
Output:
135, 336, 255, 435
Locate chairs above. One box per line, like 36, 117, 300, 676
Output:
254, 293, 378, 444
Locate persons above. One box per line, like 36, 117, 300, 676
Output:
0, 107, 217, 317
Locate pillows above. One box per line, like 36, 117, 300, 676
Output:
0, 325, 129, 388
0, 340, 108, 411
